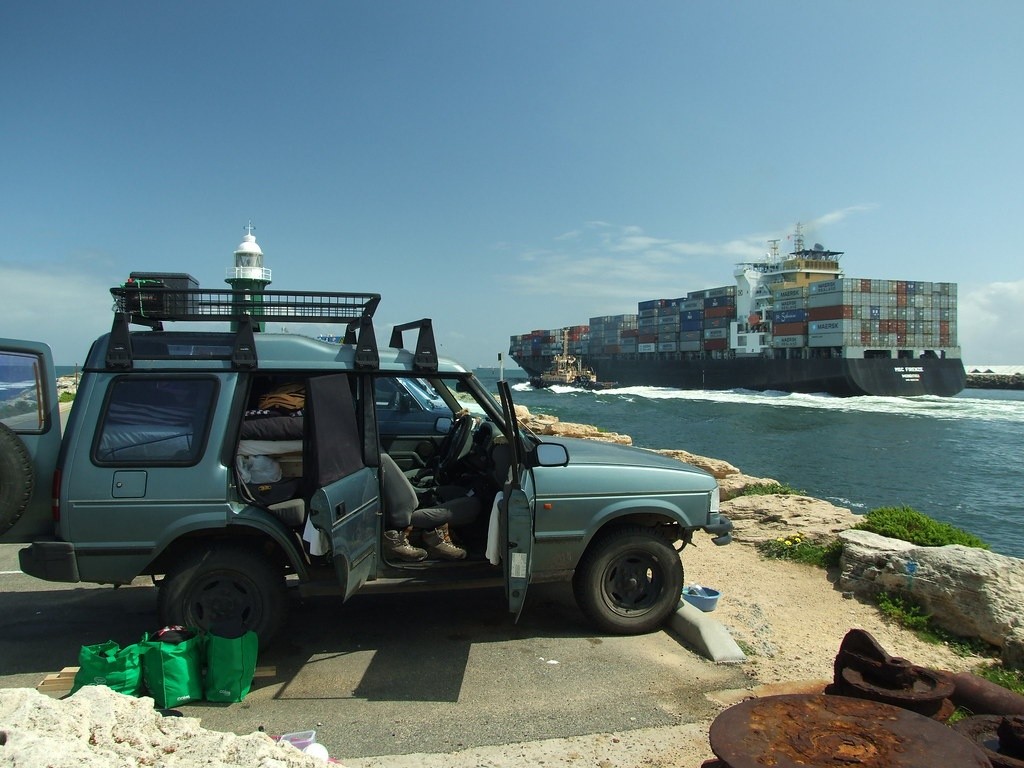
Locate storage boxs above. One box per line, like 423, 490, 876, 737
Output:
126, 283, 170, 315
130, 271, 200, 315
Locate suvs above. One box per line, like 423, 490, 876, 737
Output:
1, 291, 734, 663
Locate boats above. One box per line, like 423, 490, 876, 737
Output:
530, 327, 618, 392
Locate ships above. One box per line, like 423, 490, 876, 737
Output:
504, 221, 969, 396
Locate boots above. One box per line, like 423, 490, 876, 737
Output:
381, 527, 427, 563
423, 523, 466, 558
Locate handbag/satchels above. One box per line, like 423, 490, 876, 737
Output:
75, 639, 141, 697
257, 381, 306, 411
140, 625, 201, 708
205, 622, 258, 704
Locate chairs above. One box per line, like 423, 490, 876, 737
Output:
402, 468, 442, 500
379, 445, 487, 528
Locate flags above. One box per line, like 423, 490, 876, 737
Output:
786, 235, 790, 240
